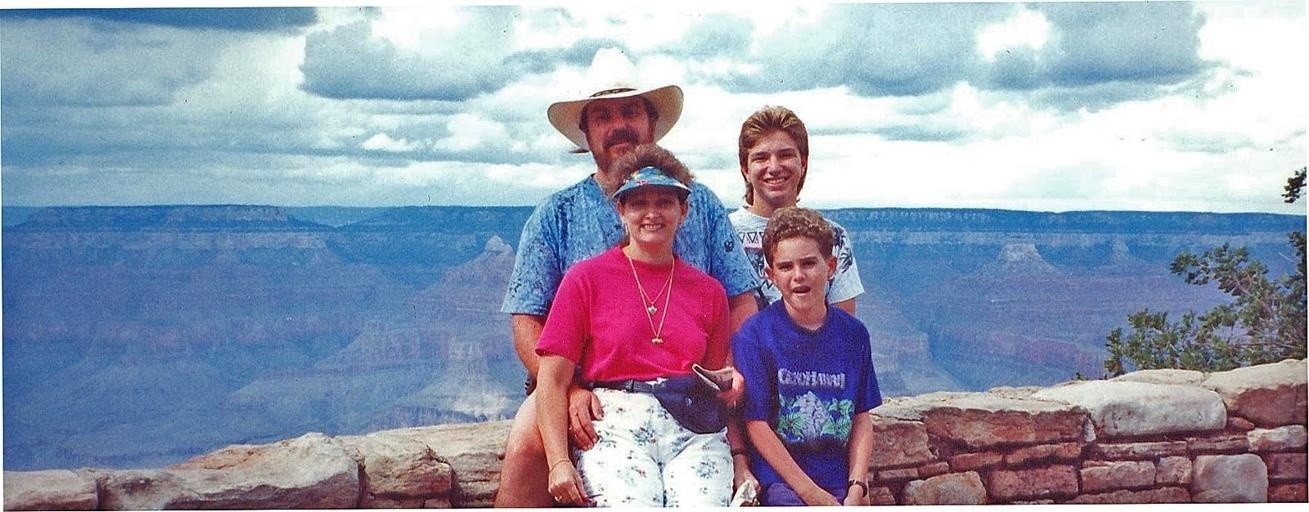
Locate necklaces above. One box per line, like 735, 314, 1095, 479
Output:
628, 247, 674, 316
626, 245, 674, 346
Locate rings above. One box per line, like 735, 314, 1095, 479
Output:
732, 400, 739, 409
567, 424, 573, 432
554, 495, 563, 503
754, 498, 760, 504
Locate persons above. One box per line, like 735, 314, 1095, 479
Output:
728, 204, 882, 507
491, 46, 759, 505
725, 102, 865, 320
534, 141, 762, 507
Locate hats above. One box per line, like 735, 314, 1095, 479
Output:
612, 166, 692, 202
548, 48, 682, 150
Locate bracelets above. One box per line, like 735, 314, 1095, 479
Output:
547, 456, 571, 474
849, 479, 869, 499
732, 448, 752, 460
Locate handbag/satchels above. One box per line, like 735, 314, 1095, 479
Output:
653, 374, 729, 434
